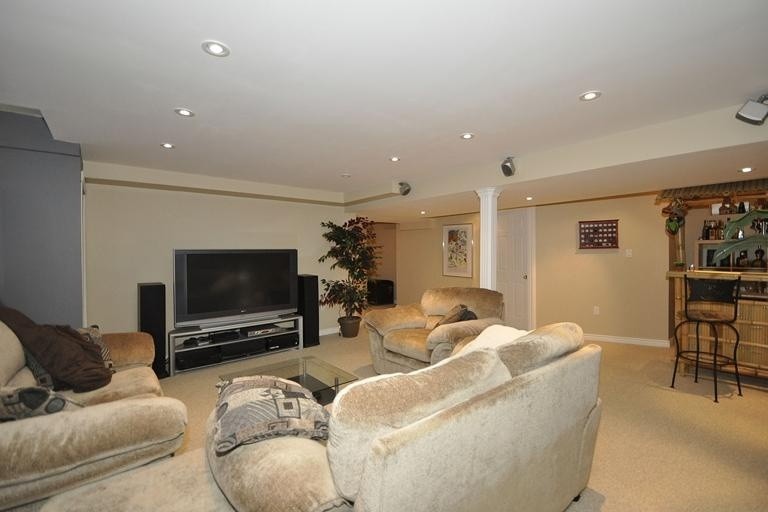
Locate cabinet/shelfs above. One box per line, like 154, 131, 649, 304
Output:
167, 315, 304, 377
666, 192, 768, 393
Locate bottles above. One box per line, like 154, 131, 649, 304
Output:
718, 221, 725, 239
738, 224, 744, 239
708, 220, 717, 240
703, 220, 709, 240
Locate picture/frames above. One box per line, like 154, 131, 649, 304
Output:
441, 222, 473, 279
576, 217, 621, 254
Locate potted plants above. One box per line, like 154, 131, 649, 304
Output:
317, 217, 386, 338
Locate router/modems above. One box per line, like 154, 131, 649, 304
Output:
211, 331, 239, 341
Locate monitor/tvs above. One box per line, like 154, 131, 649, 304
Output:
173, 249, 298, 332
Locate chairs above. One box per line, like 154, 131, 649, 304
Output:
671, 274, 743, 402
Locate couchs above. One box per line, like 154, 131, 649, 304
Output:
363, 286, 504, 375
204, 324, 605, 511
0, 305, 189, 511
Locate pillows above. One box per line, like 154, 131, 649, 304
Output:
0, 386, 82, 422
433, 304, 468, 326
25, 325, 116, 390
327, 348, 514, 503
213, 375, 330, 456
496, 322, 586, 378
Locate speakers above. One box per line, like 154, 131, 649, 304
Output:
501, 159, 515, 177
399, 182, 411, 197
735, 94, 768, 126
298, 274, 320, 348
137, 282, 168, 379
